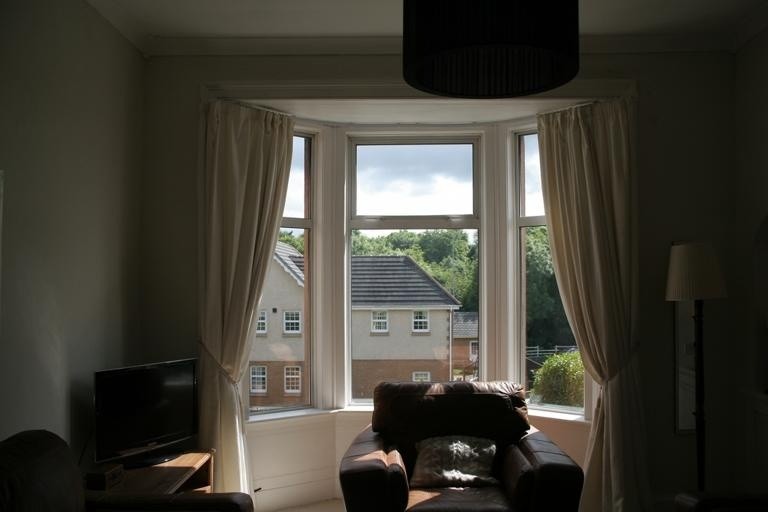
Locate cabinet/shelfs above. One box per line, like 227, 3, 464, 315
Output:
86, 447, 215, 495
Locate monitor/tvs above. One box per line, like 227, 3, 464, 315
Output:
93, 358, 198, 469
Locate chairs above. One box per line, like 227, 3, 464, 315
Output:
0, 429, 254, 512
339, 382, 584, 511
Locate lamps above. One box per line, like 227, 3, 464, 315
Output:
664, 241, 729, 495
402, 0, 581, 100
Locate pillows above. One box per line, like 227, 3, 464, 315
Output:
409, 435, 513, 488
